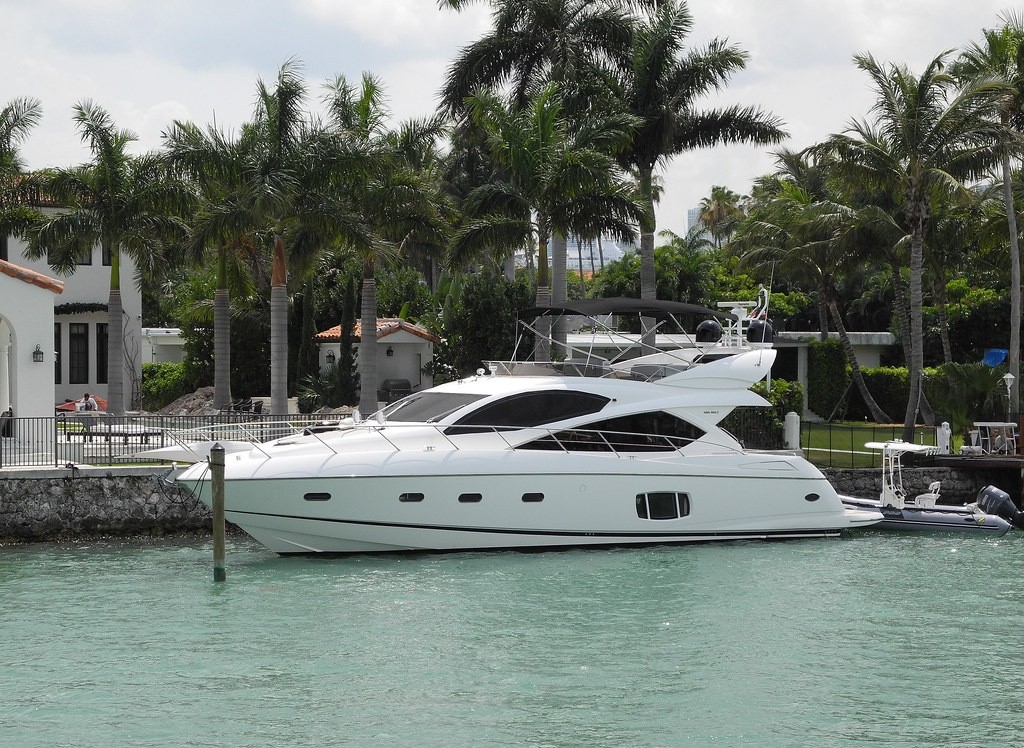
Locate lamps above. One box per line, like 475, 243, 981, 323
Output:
32, 344, 43, 362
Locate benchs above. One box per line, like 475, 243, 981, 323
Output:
383, 378, 412, 396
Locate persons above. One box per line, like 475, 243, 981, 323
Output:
1, 407, 12, 437
80, 393, 97, 411
993, 430, 1014, 455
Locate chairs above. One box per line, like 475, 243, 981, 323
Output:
219, 399, 263, 425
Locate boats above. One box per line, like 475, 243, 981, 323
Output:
838, 439, 1024, 540
166, 295, 887, 554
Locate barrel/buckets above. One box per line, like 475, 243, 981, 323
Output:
75, 403, 86, 413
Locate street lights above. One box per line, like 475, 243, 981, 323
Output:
1003, 372, 1015, 422
